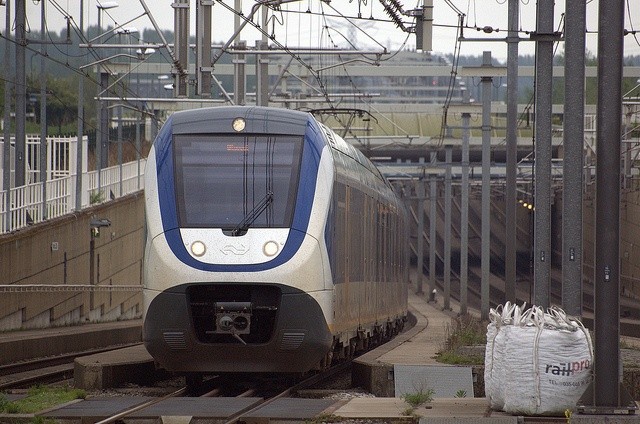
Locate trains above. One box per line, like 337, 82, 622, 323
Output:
140, 105, 412, 376
